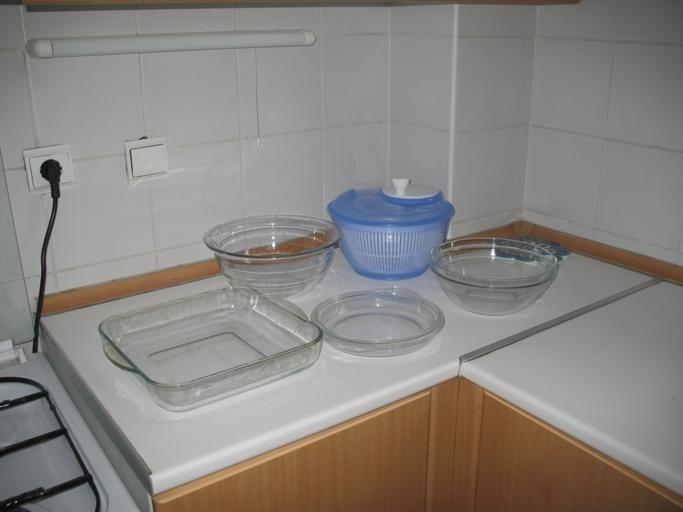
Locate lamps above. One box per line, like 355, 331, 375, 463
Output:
29, 30, 316, 58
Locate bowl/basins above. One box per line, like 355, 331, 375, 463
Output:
202, 214, 344, 301
427, 236, 559, 316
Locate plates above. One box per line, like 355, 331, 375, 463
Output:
97, 283, 323, 414
309, 284, 446, 357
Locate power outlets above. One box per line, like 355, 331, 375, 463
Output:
22, 144, 75, 194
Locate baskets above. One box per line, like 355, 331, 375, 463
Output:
335, 219, 445, 274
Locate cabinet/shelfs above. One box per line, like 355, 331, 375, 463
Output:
454, 375, 683, 511
153, 373, 457, 512
